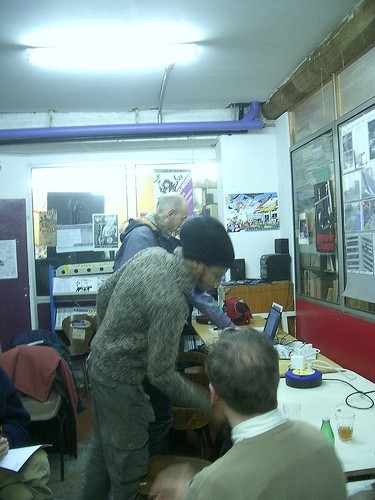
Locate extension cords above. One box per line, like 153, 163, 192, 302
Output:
285, 368, 322, 388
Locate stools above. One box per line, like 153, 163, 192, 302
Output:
137, 456, 212, 495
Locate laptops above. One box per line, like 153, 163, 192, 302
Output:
263, 307, 282, 341
254, 302, 283, 339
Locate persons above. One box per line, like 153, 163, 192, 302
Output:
81, 216, 235, 500
177, 325, 350, 500
0, 366, 51, 500
112, 190, 240, 458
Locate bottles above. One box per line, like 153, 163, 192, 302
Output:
319, 416, 334, 450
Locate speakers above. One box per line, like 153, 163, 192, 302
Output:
230, 259, 245, 281
275, 239, 289, 252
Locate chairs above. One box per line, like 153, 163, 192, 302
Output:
167, 350, 212, 459
0, 314, 96, 480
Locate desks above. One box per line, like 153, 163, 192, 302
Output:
224, 280, 294, 314
190, 319, 375, 477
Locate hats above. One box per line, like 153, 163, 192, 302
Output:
179, 215, 234, 269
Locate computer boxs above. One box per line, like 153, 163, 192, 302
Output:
259, 254, 291, 282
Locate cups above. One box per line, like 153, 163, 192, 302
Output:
335, 411, 355, 442
283, 401, 300, 421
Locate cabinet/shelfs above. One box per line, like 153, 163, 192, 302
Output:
48, 261, 115, 333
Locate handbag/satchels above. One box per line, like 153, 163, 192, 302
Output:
222, 296, 254, 325
